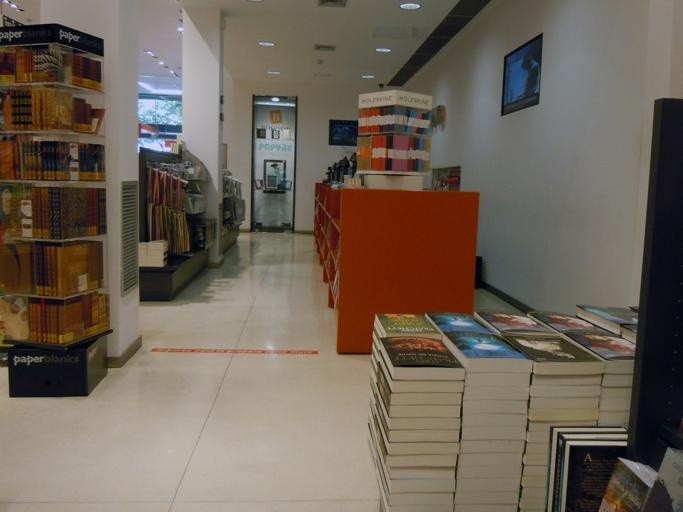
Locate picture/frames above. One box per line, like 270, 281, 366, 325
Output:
256, 159, 292, 195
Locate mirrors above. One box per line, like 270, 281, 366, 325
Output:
250, 94, 297, 234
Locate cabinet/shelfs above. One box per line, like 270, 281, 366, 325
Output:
217, 174, 244, 255
313, 91, 480, 354
140, 147, 212, 304
1, 24, 116, 399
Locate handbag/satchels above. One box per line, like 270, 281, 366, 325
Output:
146, 166, 191, 256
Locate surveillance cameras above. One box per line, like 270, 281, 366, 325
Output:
377, 83, 384, 89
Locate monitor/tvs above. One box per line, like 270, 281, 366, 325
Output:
329, 119, 358, 146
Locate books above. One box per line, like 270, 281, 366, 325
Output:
364, 300, 639, 512
597, 446, 683, 511
0, 40, 111, 350
